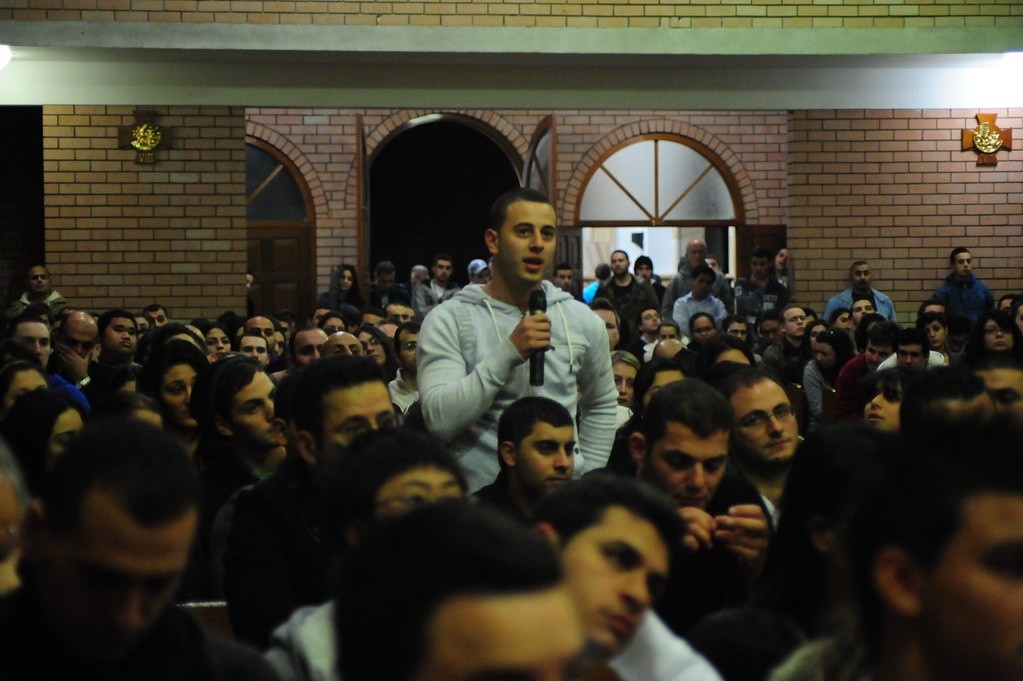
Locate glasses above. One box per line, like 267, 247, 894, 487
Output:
360, 337, 382, 349
400, 341, 418, 350
730, 406, 795, 428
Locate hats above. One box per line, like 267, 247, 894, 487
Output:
467, 259, 488, 275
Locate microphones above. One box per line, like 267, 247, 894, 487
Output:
528, 288, 548, 386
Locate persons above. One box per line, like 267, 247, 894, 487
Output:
531, 472, 687, 658
590, 380, 805, 681
775, 427, 884, 641
0, 418, 280, 681
775, 435, 929, 680
190, 355, 285, 558
861, 418, 1023, 681
0, 388, 84, 506
226, 358, 398, 648
0, 439, 27, 596
719, 369, 798, 526
263, 426, 469, 680
471, 396, 576, 530
336, 500, 586, 681
1, 238, 1023, 434
138, 339, 209, 458
933, 246, 994, 323
415, 186, 619, 494
84, 391, 163, 430
823, 261, 897, 323
